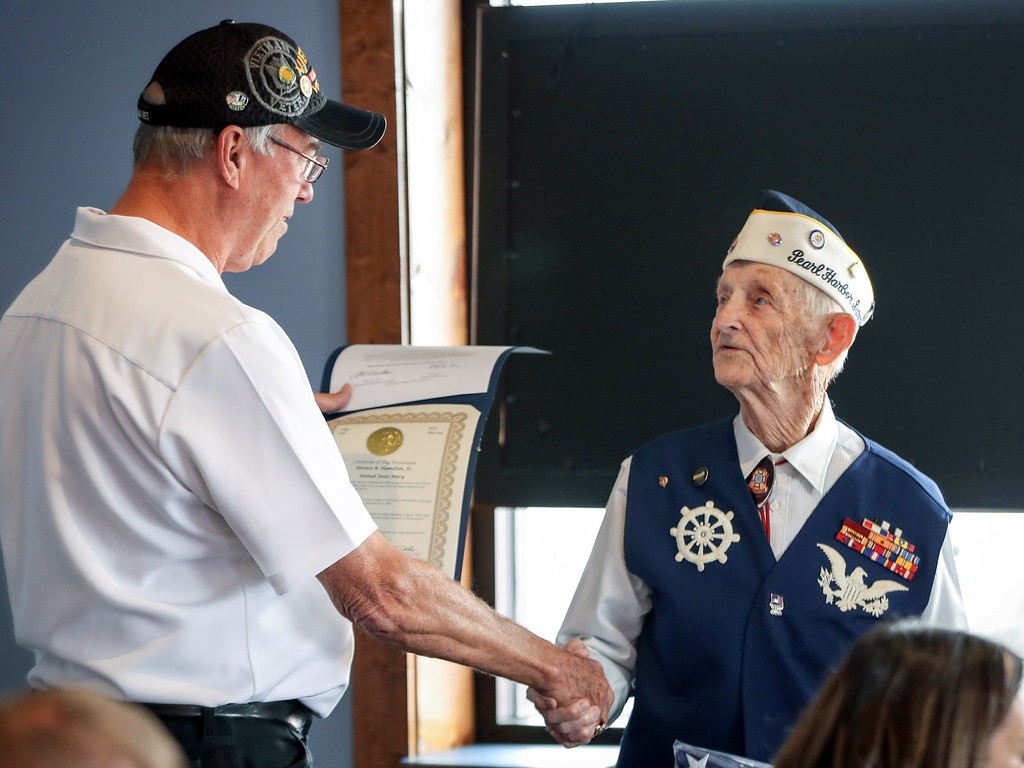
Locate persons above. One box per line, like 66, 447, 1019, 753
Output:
527, 188, 970, 768
777, 622, 1024, 768
0, 686, 181, 768
0, 14, 617, 768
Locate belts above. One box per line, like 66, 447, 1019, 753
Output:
143, 699, 312, 741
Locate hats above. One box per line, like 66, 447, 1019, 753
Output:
722, 190, 875, 326
136, 19, 387, 151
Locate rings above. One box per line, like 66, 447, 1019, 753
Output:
595, 722, 607, 735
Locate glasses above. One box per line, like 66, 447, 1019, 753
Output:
268, 134, 330, 184
